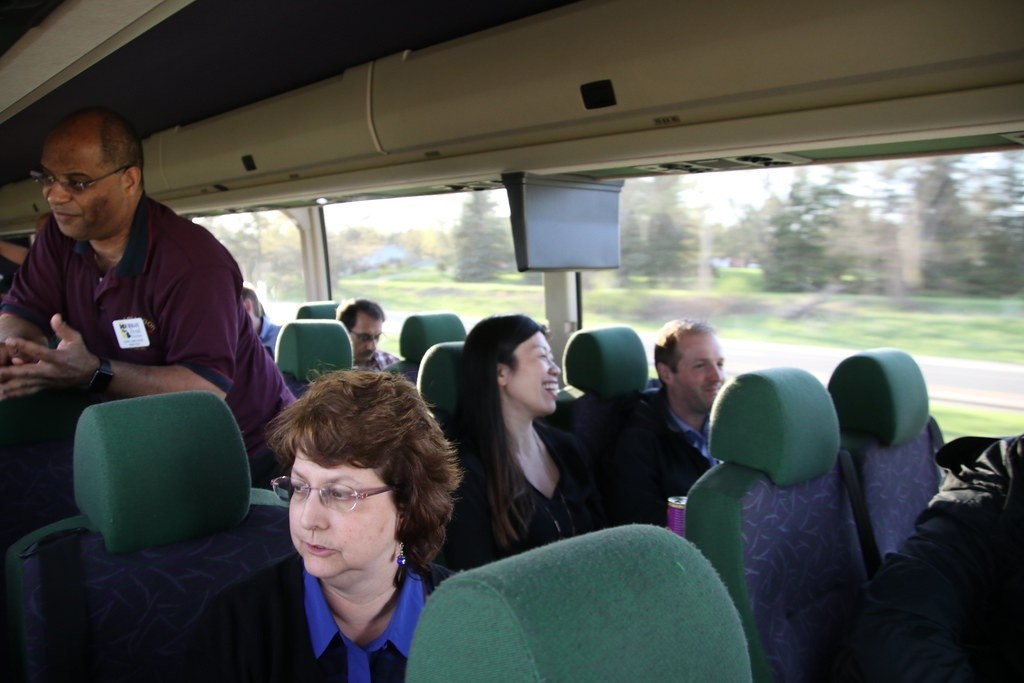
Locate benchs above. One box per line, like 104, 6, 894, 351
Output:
0, 300, 946, 683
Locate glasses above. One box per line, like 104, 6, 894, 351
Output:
270, 475, 395, 512
349, 330, 383, 341
29, 165, 128, 190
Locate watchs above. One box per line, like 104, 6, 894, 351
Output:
83, 357, 114, 394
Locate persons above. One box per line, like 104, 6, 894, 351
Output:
609, 318, 724, 527
185, 371, 460, 683
847, 434, 1024, 683
447, 315, 608, 565
0, 213, 51, 264
335, 299, 399, 372
242, 287, 281, 361
0, 108, 298, 492
